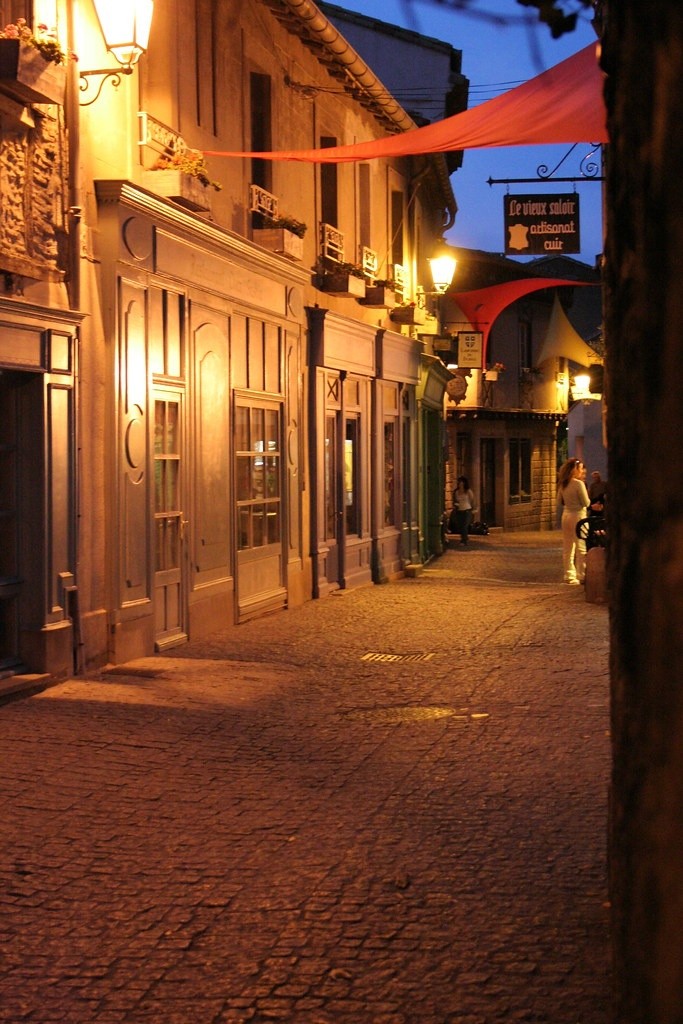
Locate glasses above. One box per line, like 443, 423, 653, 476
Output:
575, 460, 580, 464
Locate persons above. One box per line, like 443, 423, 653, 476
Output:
556, 459, 608, 585
452, 477, 477, 547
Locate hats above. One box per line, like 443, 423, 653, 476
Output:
591, 470, 600, 475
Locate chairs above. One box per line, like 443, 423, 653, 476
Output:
576, 515, 607, 553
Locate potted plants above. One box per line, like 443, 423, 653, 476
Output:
521, 367, 544, 383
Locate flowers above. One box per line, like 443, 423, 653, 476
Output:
332, 261, 366, 280
264, 214, 307, 239
425, 311, 434, 320
438, 331, 457, 340
154, 152, 223, 193
490, 362, 507, 373
0, 17, 79, 67
400, 298, 427, 309
373, 279, 395, 292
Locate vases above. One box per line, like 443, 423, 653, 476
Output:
148, 170, 212, 213
417, 320, 441, 336
486, 371, 505, 382
0, 38, 67, 105
361, 287, 395, 309
433, 338, 456, 351
392, 307, 425, 325
328, 274, 365, 298
252, 228, 304, 261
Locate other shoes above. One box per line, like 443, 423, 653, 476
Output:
457, 541, 461, 545
567, 577, 580, 584
576, 574, 585, 580
459, 542, 465, 547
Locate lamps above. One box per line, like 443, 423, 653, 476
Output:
417, 237, 460, 307
564, 369, 592, 394
79, 0, 157, 106
